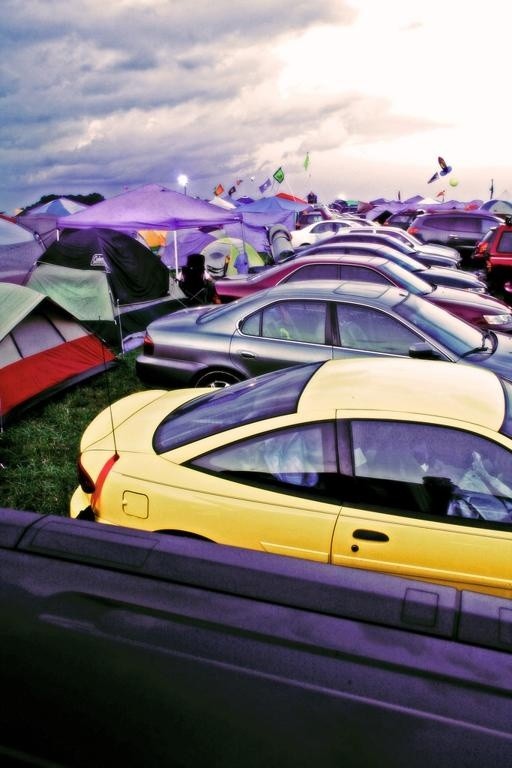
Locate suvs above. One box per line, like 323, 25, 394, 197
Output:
294, 209, 324, 230
407, 212, 507, 256
485, 224, 511, 294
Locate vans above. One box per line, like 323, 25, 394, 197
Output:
471, 227, 499, 267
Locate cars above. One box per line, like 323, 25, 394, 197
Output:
214, 253, 512, 329
325, 226, 462, 262
299, 232, 458, 269
383, 210, 424, 232
69, 357, 511, 600
290, 219, 364, 250
132, 278, 512, 383
278, 241, 488, 294
